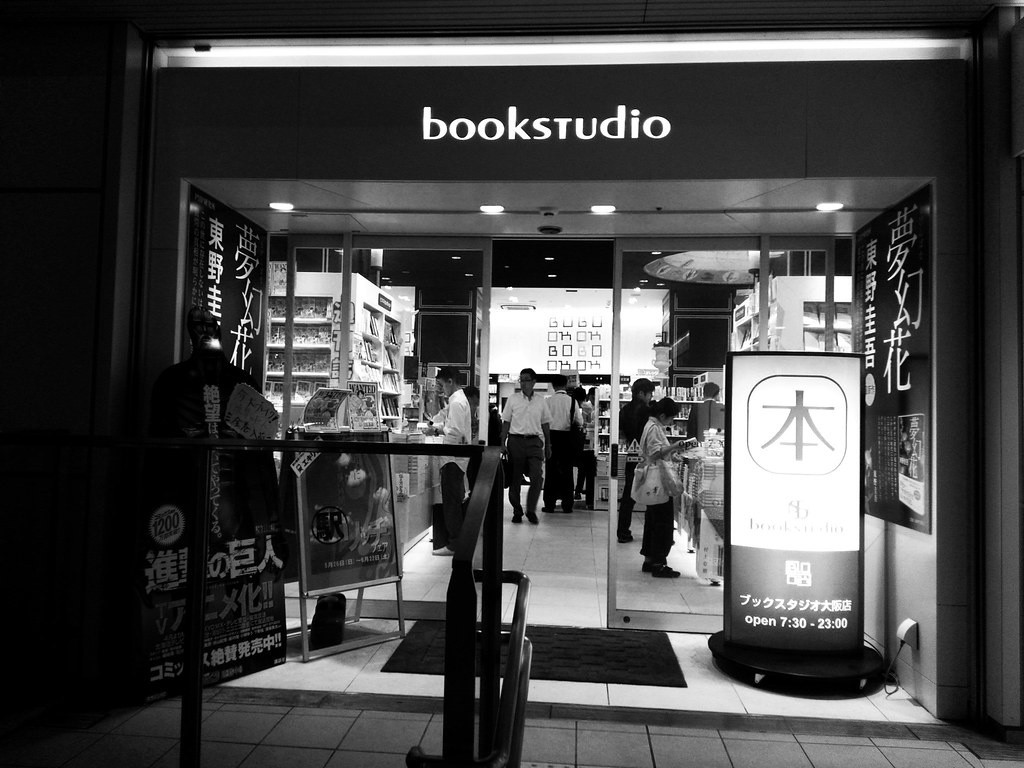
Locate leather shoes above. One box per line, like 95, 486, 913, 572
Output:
512, 512, 524, 523
525, 512, 539, 524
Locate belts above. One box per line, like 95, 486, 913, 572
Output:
511, 434, 539, 439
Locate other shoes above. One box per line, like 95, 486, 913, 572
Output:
585, 505, 593, 510
542, 507, 554, 513
642, 561, 681, 578
575, 491, 581, 499
563, 511, 571, 513
617, 530, 633, 542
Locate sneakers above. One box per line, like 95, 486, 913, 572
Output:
431, 546, 454, 556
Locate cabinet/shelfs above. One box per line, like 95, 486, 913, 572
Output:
267, 292, 402, 428
488, 276, 854, 585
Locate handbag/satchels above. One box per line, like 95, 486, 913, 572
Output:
568, 397, 586, 468
659, 448, 684, 496
630, 424, 669, 505
500, 452, 510, 488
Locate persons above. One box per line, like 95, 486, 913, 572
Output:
421, 366, 472, 557
617, 377, 657, 543
639, 398, 687, 578
568, 384, 606, 511
540, 373, 584, 513
686, 382, 725, 444
499, 368, 554, 524
463, 385, 479, 499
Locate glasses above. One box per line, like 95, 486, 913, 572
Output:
518, 378, 533, 383
